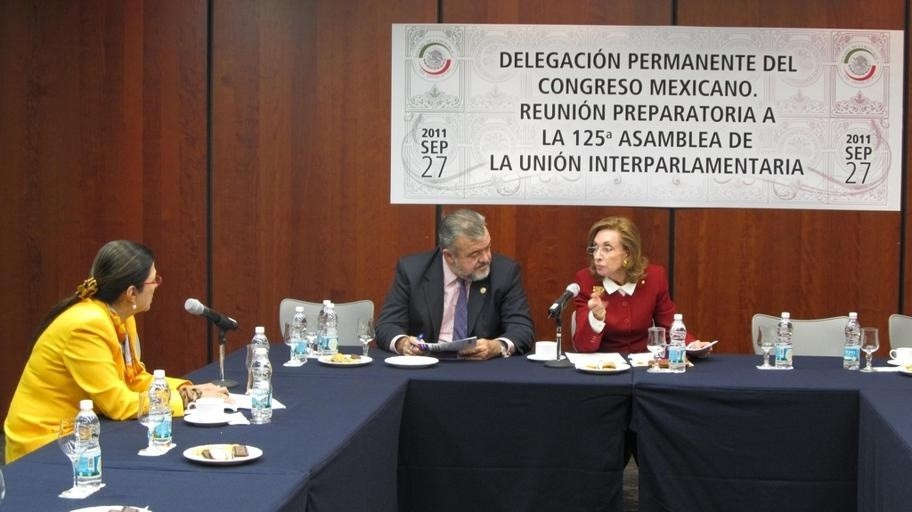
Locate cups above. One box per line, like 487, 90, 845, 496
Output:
889, 346, 912, 361
0, 470, 7, 503
244, 342, 255, 394
185, 396, 226, 420
535, 341, 558, 357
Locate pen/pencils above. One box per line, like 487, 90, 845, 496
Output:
407, 334, 424, 352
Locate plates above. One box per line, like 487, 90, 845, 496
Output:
383, 355, 441, 369
317, 354, 374, 367
181, 442, 266, 468
887, 359, 912, 365
526, 353, 566, 362
896, 365, 912, 375
65, 503, 153, 512
574, 359, 632, 375
183, 414, 233, 426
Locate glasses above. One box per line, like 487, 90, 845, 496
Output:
130, 275, 162, 287
585, 245, 614, 256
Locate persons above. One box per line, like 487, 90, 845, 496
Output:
369, 208, 534, 361
4, 238, 233, 467
571, 216, 714, 360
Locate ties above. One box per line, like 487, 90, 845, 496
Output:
453, 278, 468, 342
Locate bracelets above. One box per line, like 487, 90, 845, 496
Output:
191, 388, 202, 401
183, 386, 194, 409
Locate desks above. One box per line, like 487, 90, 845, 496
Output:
1, 457, 312, 512
856, 370, 912, 512
222, 338, 645, 511
0, 361, 409, 512
632, 350, 901, 511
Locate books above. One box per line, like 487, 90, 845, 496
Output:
684, 339, 719, 354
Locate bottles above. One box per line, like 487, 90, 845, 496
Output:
291, 305, 308, 365
669, 312, 687, 374
318, 298, 340, 355
842, 312, 862, 373
251, 347, 274, 426
252, 325, 270, 350
75, 399, 103, 489
146, 366, 175, 449
777, 311, 795, 370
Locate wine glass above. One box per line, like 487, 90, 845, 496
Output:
859, 327, 880, 373
647, 326, 667, 376
304, 319, 318, 359
283, 320, 304, 368
756, 325, 777, 371
356, 318, 376, 356
135, 389, 165, 458
58, 415, 93, 498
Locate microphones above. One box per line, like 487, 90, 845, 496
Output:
548, 283, 580, 316
183, 297, 237, 329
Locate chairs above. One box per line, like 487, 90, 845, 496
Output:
750, 313, 863, 358
886, 311, 911, 354
274, 294, 375, 350
